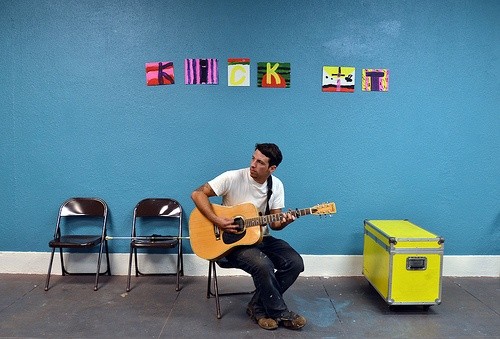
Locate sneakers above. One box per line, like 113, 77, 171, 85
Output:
277, 312, 307, 330
247, 306, 278, 329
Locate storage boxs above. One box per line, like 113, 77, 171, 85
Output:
361, 217, 445, 308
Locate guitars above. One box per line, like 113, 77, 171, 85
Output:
188, 201, 337, 261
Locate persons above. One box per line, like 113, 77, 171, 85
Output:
190, 141, 306, 332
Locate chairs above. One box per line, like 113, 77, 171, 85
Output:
207, 256, 257, 319
45, 198, 111, 291
126, 198, 184, 293
